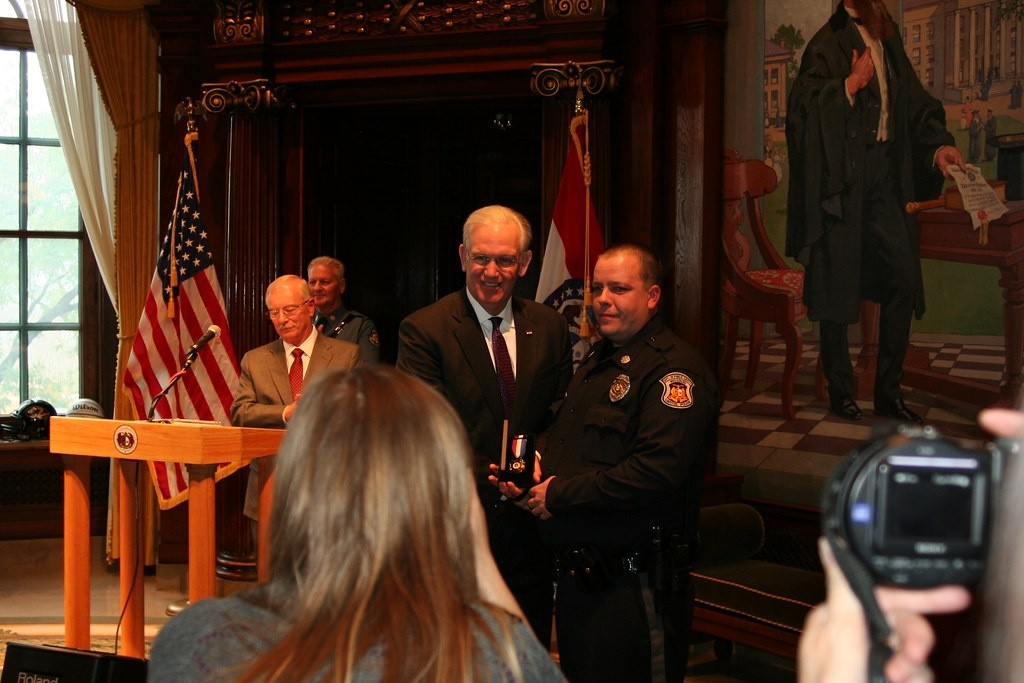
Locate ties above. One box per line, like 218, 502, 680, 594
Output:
289, 348, 304, 402
488, 317, 515, 432
317, 320, 327, 333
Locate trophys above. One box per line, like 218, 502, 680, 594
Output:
495, 417, 535, 487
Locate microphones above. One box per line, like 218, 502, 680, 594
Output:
186, 325, 222, 356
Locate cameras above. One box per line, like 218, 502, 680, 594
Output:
821, 417, 1021, 589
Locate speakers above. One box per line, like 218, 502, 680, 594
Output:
0, 641, 148, 683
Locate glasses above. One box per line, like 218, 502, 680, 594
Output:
265, 300, 309, 319
466, 255, 520, 265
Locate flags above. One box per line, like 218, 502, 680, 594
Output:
533, 109, 606, 376
118, 151, 261, 508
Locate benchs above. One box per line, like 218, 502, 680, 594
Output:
691, 500, 826, 661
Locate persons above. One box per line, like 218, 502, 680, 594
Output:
518, 245, 719, 683
306, 257, 380, 371
145, 368, 569, 683
389, 205, 575, 651
795, 391, 1024, 683
230, 275, 365, 553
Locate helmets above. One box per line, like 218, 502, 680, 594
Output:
1, 399, 57, 438
65, 398, 105, 418
